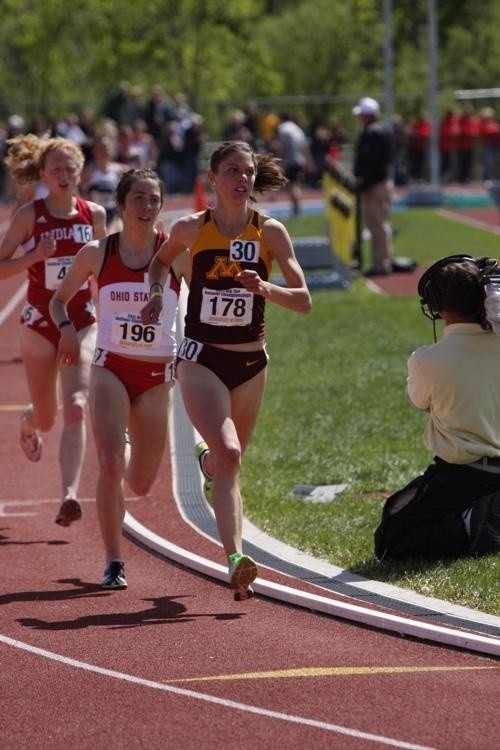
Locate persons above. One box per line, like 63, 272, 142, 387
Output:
0, 134, 108, 527
373, 254, 500, 560
48, 167, 192, 589
140, 140, 312, 602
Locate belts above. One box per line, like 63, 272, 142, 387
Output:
474, 456, 500, 467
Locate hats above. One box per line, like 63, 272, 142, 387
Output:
352, 97, 380, 116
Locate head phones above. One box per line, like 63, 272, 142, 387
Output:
417, 253, 480, 321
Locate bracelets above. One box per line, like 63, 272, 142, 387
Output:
58, 320, 72, 329
150, 282, 164, 294
151, 292, 162, 300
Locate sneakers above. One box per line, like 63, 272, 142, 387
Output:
55, 492, 82, 527
469, 494, 498, 556
229, 554, 259, 601
20, 404, 42, 462
195, 441, 216, 508
101, 559, 128, 589
